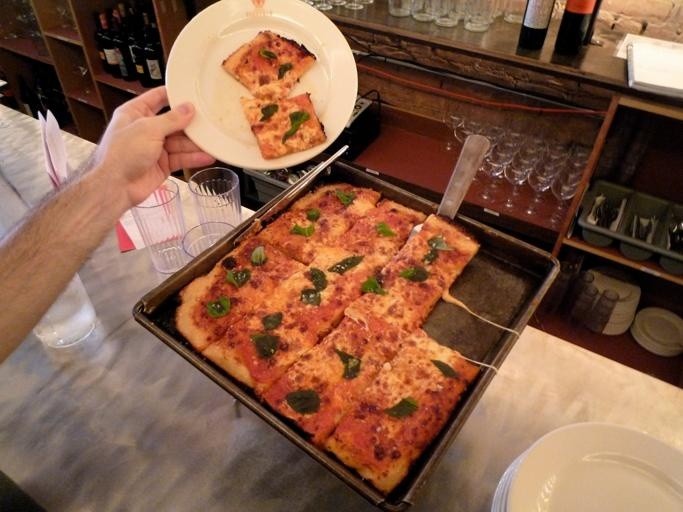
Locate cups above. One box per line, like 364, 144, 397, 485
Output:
182, 165, 242, 260
31, 271, 97, 349
130, 178, 195, 273
565, 270, 619, 336
387, 0, 528, 34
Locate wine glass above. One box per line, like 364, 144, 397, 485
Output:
444, 109, 591, 225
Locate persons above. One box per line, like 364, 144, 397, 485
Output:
0, 84, 218, 364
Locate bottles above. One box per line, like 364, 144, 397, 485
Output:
16, 66, 72, 129
518, 0, 557, 52
94, 2, 166, 88
554, 0, 604, 57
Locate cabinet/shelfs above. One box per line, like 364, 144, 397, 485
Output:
0, 1, 681, 395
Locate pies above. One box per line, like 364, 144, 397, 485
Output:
221, 29, 316, 101
175, 183, 480, 497
239, 93, 326, 160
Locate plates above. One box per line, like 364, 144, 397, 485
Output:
166, 0, 360, 171
632, 308, 682, 358
491, 422, 682, 512
585, 264, 642, 336
